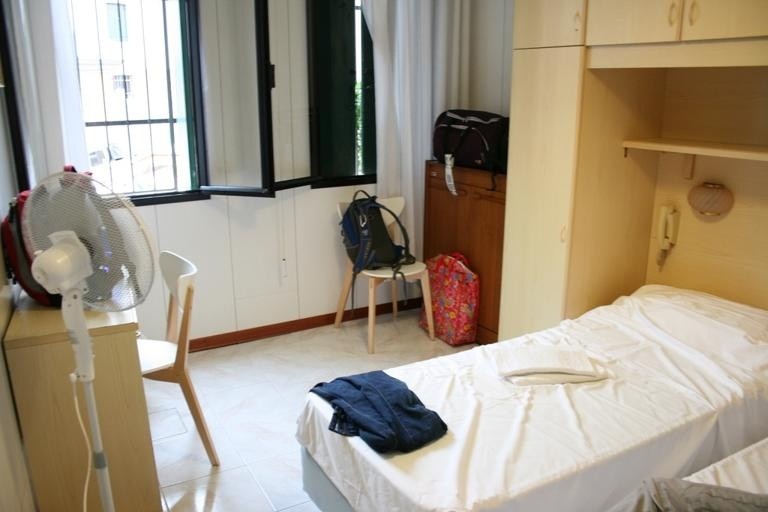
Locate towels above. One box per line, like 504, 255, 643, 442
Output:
500, 343, 610, 384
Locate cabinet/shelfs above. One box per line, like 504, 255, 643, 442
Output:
498, 47, 664, 343
3, 263, 163, 511
512, 0, 587, 50
423, 160, 506, 344
586, 0, 767, 47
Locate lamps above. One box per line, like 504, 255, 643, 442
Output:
687, 182, 734, 217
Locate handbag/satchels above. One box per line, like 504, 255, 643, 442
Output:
420, 252, 481, 347
434, 108, 508, 173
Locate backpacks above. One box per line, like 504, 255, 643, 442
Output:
2, 164, 143, 304
339, 190, 416, 273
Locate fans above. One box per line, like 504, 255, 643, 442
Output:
21, 172, 155, 511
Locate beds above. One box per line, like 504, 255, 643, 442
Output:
296, 284, 768, 512
681, 437, 768, 494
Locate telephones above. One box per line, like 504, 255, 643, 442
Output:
658, 206, 678, 249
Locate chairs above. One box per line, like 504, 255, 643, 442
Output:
333, 197, 435, 354
136, 250, 220, 468
